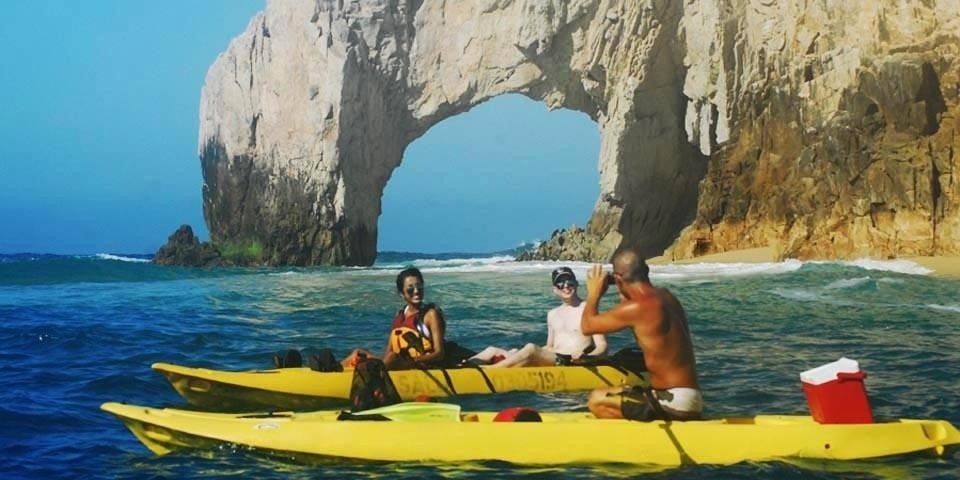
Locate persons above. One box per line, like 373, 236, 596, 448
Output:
580, 247, 704, 421
460, 266, 609, 370
350, 265, 448, 371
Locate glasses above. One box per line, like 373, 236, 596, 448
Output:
405, 285, 424, 295
556, 280, 574, 289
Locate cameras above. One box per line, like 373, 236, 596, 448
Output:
607, 273, 616, 284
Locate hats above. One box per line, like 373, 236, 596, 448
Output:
552, 266, 575, 284
